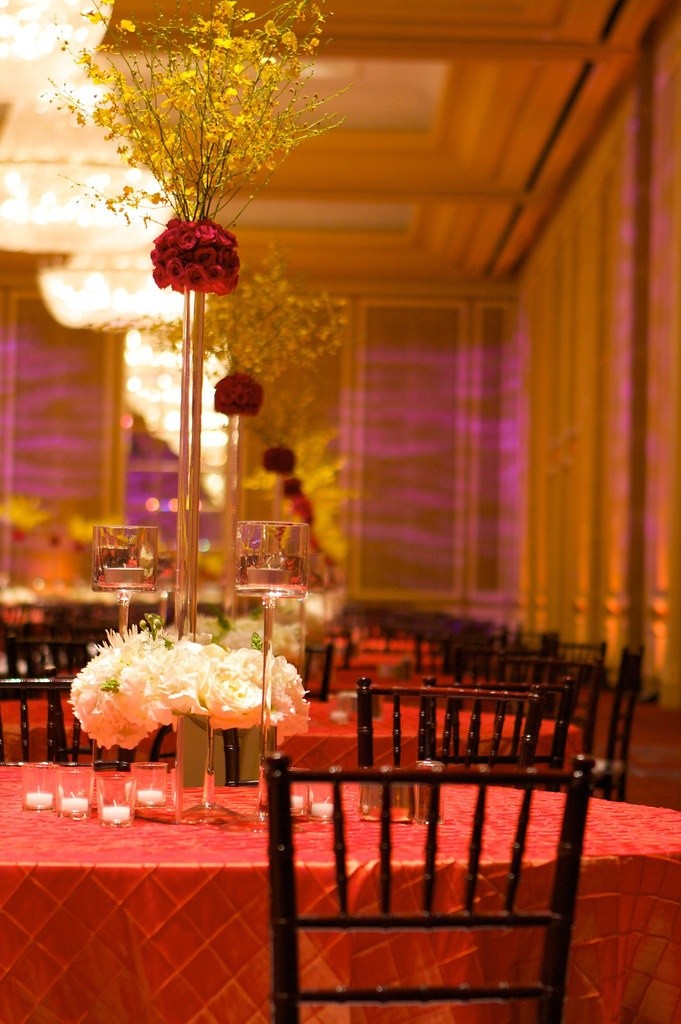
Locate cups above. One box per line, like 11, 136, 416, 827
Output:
389, 783, 419, 827
130, 763, 168, 808
287, 767, 312, 822
357, 781, 382, 822
22, 762, 61, 811
57, 763, 93, 822
309, 781, 333, 825
94, 772, 138, 828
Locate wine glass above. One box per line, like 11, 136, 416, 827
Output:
92, 525, 159, 643
221, 520, 310, 836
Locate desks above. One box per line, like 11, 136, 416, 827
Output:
321, 623, 381, 636
323, 637, 441, 651
0, 697, 583, 795
0, 761, 681, 1024
311, 666, 497, 690
312, 651, 444, 671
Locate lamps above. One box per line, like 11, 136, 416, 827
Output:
0, 0, 241, 502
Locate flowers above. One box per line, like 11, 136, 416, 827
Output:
282, 478, 302, 498
262, 448, 297, 476
150, 216, 240, 296
68, 611, 310, 751
212, 372, 265, 419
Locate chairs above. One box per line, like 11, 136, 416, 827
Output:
0, 603, 646, 1024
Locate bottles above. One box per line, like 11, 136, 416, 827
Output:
416, 761, 445, 825
